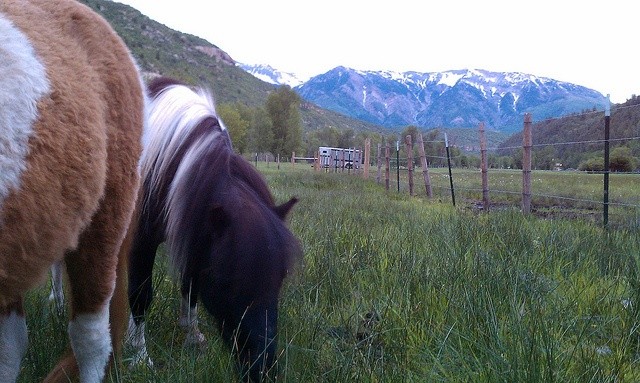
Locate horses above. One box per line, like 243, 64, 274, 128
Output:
48, 72, 305, 383
0, 0, 150, 383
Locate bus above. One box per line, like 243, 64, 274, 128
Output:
321, 148, 362, 168
